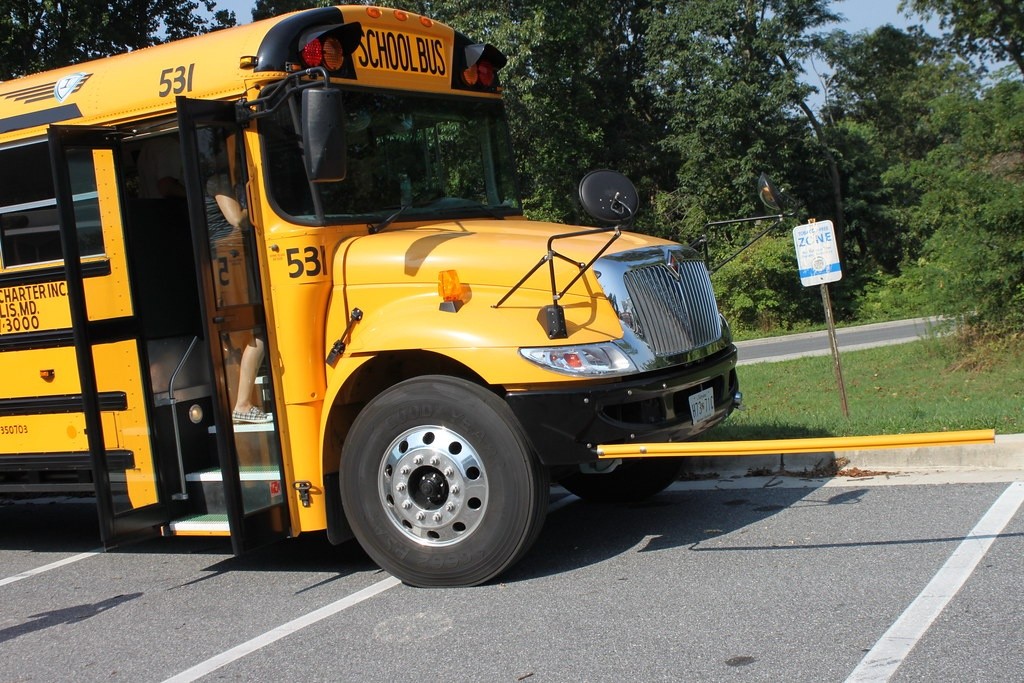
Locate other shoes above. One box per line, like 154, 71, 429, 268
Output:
233, 406, 273, 423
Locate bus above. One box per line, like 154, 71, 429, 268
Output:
0, 5, 794, 590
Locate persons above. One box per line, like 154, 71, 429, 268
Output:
204, 127, 276, 424
